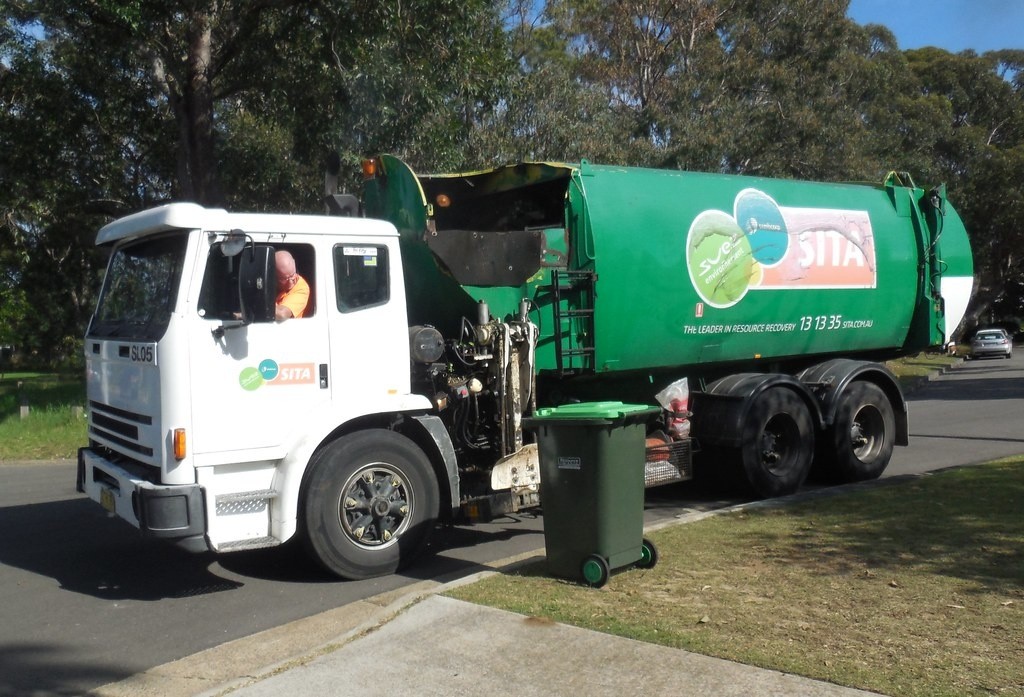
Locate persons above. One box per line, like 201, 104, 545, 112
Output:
223, 250, 310, 321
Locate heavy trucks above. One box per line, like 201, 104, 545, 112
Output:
76, 153, 973, 581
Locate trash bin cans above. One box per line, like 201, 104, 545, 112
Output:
522, 400, 657, 589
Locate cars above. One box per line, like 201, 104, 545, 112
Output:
971, 329, 1013, 360
